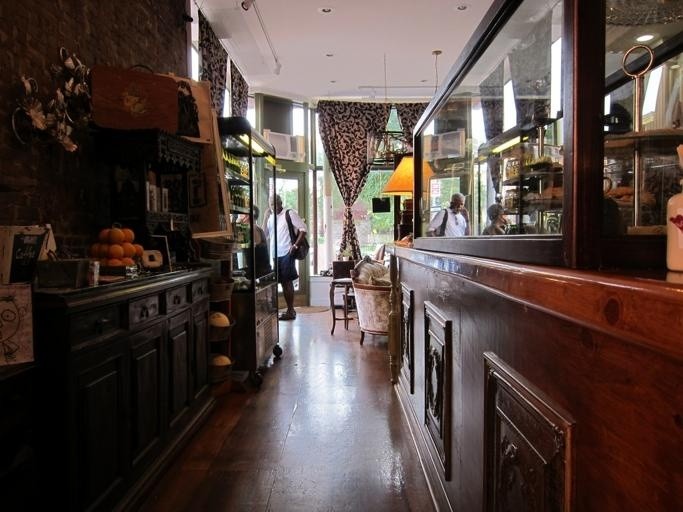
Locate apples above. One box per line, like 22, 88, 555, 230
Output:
100, 258, 108, 266
123, 243, 136, 257
109, 244, 124, 258
123, 229, 134, 243
133, 244, 144, 257
98, 243, 109, 258
98, 228, 111, 242
110, 228, 124, 243
108, 258, 121, 266
122, 258, 135, 266
90, 243, 98, 258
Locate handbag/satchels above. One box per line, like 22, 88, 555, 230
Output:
285, 210, 309, 262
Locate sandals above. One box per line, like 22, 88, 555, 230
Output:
279, 313, 295, 322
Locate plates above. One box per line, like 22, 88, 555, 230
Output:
101, 264, 140, 275
529, 198, 561, 205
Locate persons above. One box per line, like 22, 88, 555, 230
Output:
426, 193, 471, 237
261, 194, 307, 321
483, 204, 512, 235
241, 205, 268, 278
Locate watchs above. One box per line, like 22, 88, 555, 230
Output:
294, 244, 298, 249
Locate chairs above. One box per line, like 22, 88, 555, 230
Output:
350, 270, 395, 345
376, 244, 386, 262
329, 261, 356, 336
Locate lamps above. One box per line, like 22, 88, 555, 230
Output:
381, 156, 439, 249
367, 53, 413, 162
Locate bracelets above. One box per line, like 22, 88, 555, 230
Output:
466, 220, 470, 222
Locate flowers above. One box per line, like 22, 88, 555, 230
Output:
10, 47, 96, 153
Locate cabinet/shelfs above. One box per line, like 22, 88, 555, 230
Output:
412, 1, 683, 268
0, 262, 213, 511
117, 69, 203, 261
217, 117, 283, 388
